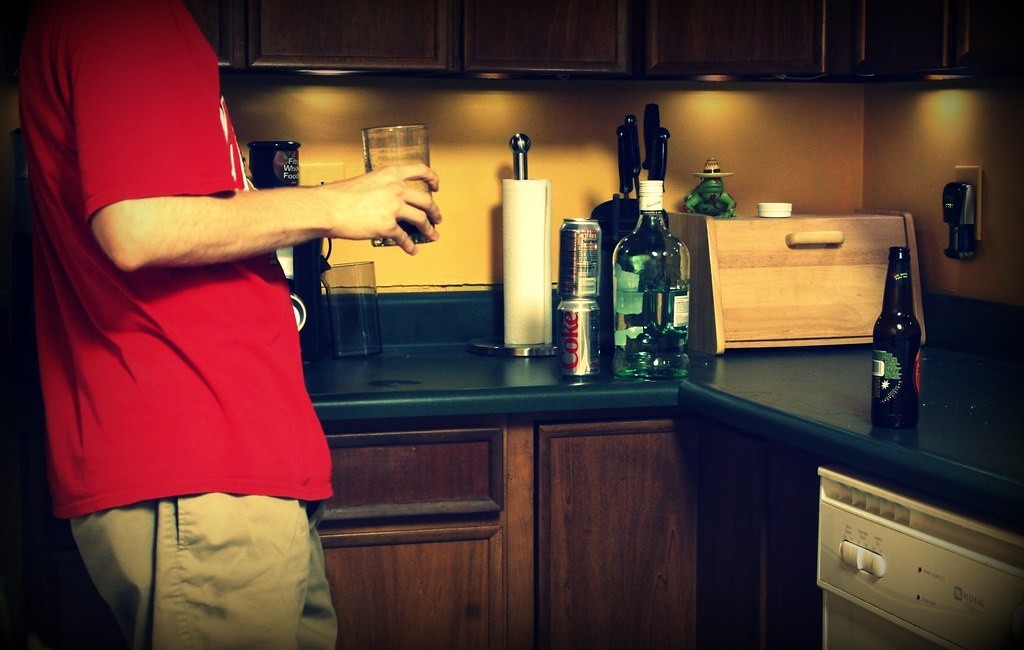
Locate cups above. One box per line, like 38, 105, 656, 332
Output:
362, 125, 435, 247
320, 261, 381, 357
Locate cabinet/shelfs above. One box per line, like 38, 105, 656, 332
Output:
314, 408, 698, 650
180, 0, 1024, 98
693, 425, 821, 650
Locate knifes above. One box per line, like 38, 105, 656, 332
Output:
617, 104, 670, 201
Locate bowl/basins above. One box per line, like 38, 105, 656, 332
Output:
757, 202, 792, 217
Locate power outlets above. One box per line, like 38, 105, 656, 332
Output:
955, 163, 983, 242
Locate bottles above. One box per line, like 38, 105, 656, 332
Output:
871, 247, 921, 431
611, 180, 690, 382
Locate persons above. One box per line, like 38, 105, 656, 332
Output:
18, 1, 442, 650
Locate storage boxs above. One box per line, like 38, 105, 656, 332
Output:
669, 209, 929, 356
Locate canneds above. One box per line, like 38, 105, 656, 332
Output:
556, 297, 601, 379
558, 218, 603, 299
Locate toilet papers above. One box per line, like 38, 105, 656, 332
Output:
501, 179, 553, 346
